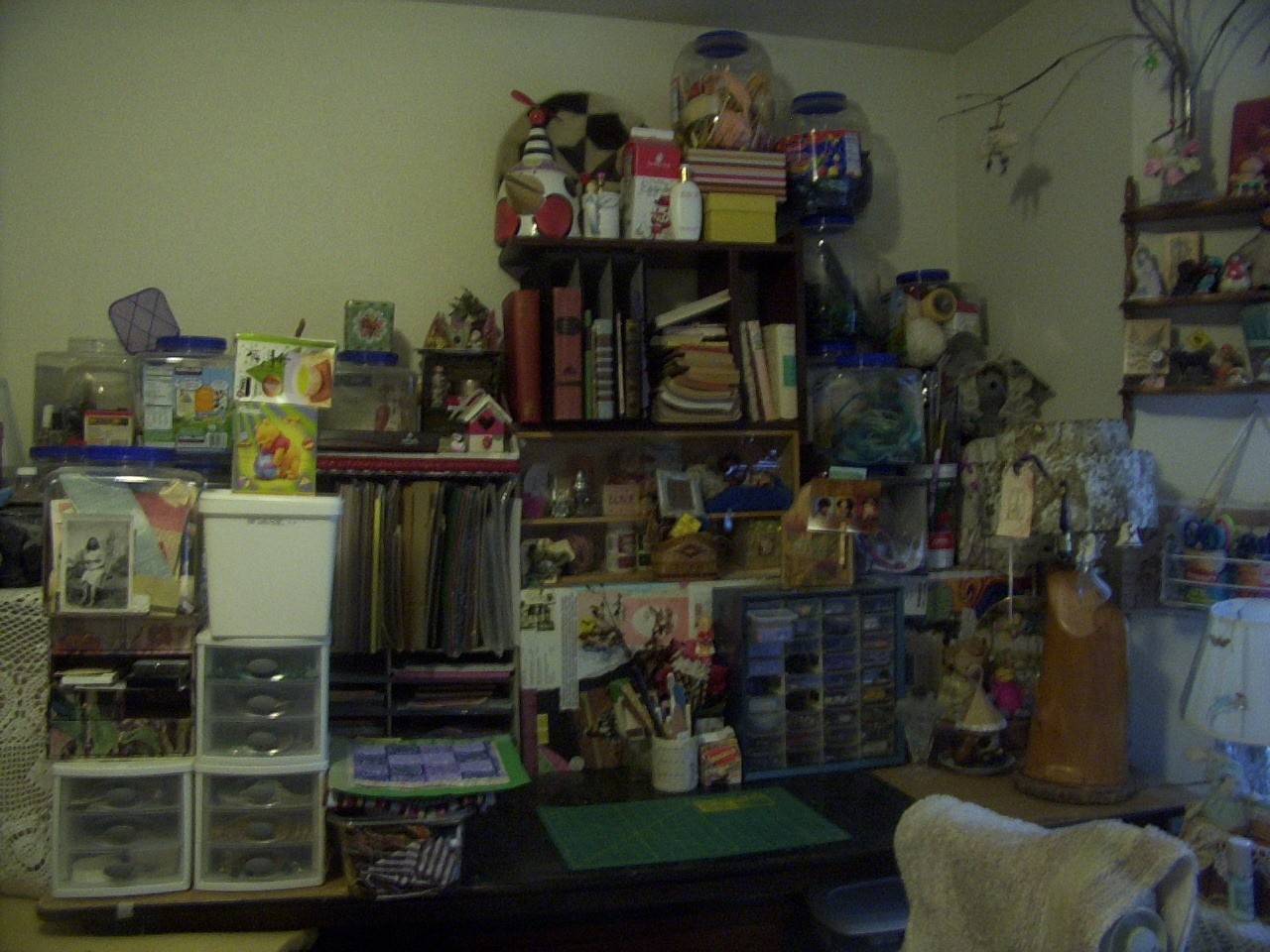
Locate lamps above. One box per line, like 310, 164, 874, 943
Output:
1177, 594, 1270, 809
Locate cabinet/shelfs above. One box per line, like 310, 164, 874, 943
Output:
30, 177, 1269, 897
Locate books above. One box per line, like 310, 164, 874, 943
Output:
328, 148, 798, 659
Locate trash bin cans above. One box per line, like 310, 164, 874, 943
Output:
811, 876, 909, 952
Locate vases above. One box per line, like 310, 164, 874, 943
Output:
1164, 86, 1217, 196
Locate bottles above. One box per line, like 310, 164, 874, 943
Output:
651, 736, 698, 793
914, 461, 959, 570
320, 348, 419, 436
671, 28, 780, 158
781, 90, 872, 216
806, 343, 926, 475
126, 335, 235, 449
884, 270, 962, 370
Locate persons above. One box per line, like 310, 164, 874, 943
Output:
861, 498, 881, 517
813, 498, 831, 519
836, 497, 855, 518
76, 537, 105, 607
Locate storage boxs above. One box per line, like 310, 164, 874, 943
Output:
44, 149, 1255, 896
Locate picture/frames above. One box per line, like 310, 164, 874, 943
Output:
58, 515, 134, 614
